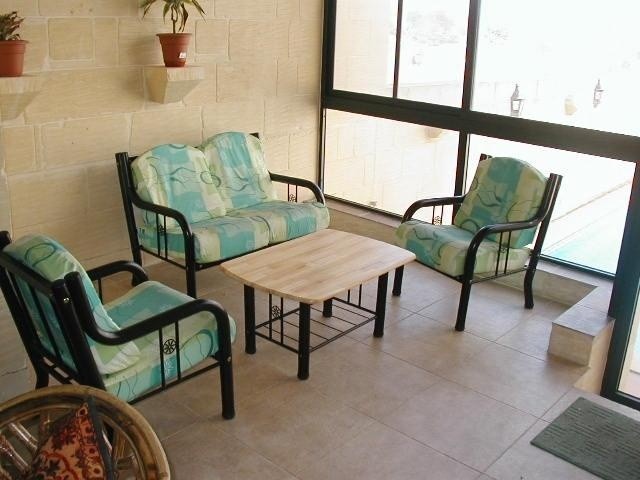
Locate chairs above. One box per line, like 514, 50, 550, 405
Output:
0, 225, 240, 461
384, 150, 566, 331
0, 380, 174, 480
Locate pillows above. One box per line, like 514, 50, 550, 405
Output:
9, 393, 117, 480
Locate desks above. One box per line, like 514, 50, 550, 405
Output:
211, 225, 420, 387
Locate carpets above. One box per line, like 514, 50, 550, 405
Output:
525, 388, 639, 480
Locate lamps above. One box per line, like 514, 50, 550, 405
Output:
592, 78, 605, 108
510, 82, 527, 117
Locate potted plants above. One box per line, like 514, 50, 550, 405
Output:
138, 0, 210, 68
0, 9, 32, 77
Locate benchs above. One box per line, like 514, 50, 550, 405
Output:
111, 128, 334, 305
